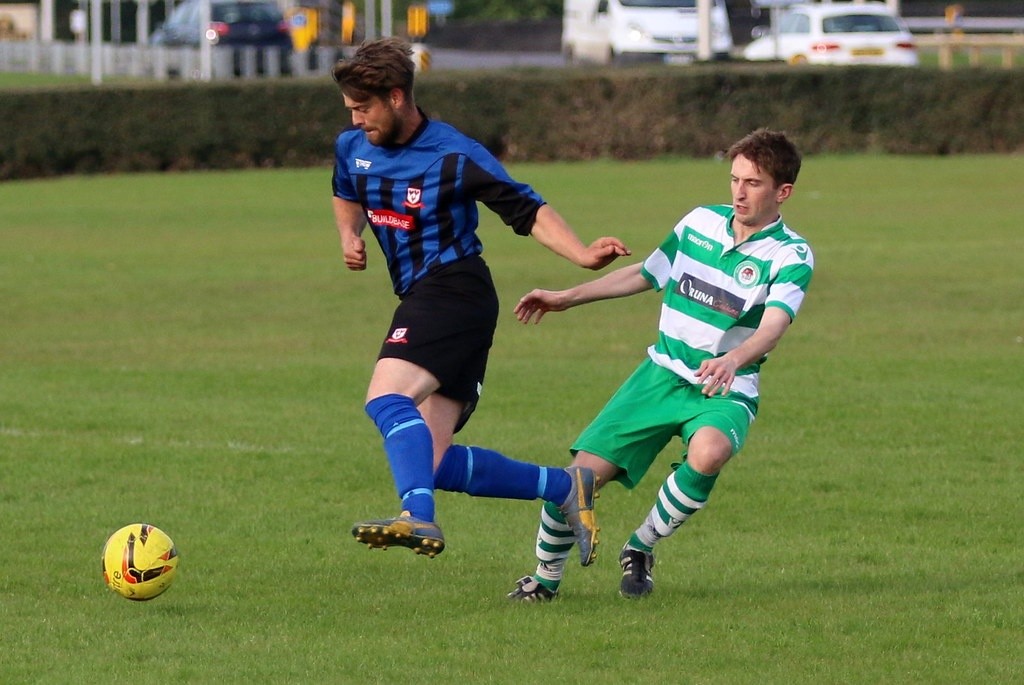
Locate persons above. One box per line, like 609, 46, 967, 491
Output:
504, 127, 812, 603
327, 35, 634, 564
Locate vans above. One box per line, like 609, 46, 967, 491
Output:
562, 0, 734, 67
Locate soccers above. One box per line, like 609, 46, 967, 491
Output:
100, 524, 180, 603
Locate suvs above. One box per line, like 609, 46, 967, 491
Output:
745, 3, 919, 67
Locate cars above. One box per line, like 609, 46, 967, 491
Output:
150, 0, 292, 47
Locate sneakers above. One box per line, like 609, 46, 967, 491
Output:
617, 538, 656, 601
351, 510, 446, 559
556, 465, 601, 567
505, 575, 561, 603
